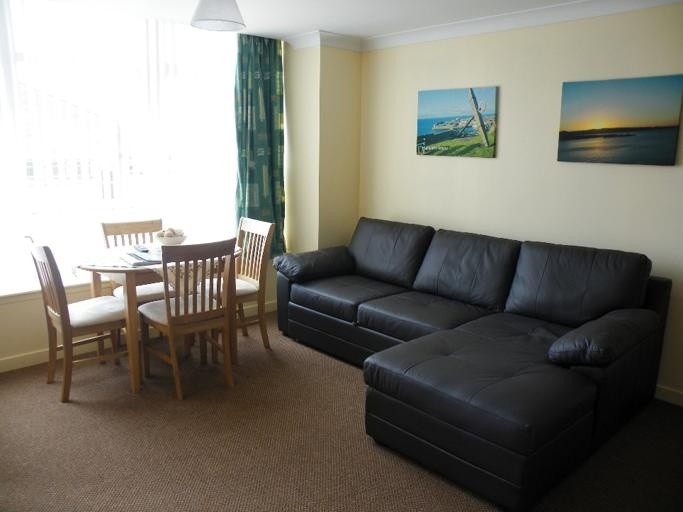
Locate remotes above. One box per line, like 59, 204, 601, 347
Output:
134, 247, 149, 253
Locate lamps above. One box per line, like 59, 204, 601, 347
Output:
189, 2, 245, 36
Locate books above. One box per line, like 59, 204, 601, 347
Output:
120, 252, 161, 266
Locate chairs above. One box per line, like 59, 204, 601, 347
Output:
22, 216, 279, 404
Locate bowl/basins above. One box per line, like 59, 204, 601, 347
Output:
156, 235, 186, 246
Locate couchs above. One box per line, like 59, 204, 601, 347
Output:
271, 213, 674, 509
